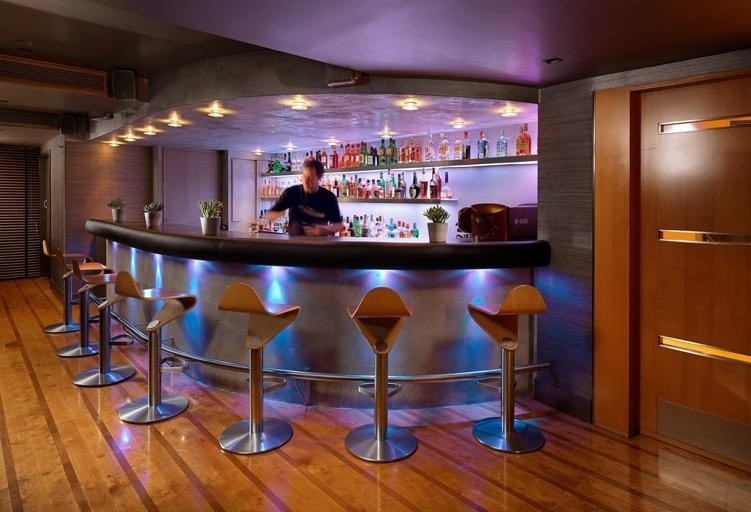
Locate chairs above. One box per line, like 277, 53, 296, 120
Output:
214, 281, 300, 456
343, 286, 419, 464
32, 237, 100, 334
51, 248, 108, 358
464, 285, 561, 456
65, 264, 135, 389
108, 268, 197, 426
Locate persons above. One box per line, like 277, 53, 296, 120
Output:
260, 158, 344, 236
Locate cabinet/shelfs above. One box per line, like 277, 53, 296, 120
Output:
259, 154, 538, 204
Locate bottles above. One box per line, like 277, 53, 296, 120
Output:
258, 209, 419, 238
265, 129, 450, 174
332, 169, 451, 199
261, 178, 300, 200
476, 131, 489, 159
453, 133, 462, 160
514, 122, 531, 156
461, 131, 471, 160
495, 130, 507, 157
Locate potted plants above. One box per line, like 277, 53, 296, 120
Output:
106, 195, 127, 223
194, 199, 223, 236
417, 203, 454, 243
141, 199, 164, 228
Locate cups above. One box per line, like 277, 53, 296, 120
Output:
248, 218, 259, 233
